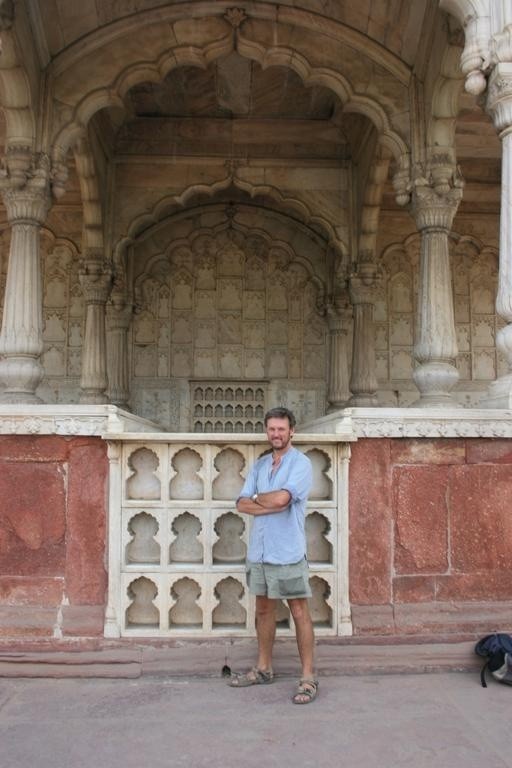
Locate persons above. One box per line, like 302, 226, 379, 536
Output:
231, 408, 317, 703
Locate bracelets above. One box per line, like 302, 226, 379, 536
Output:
252, 494, 258, 501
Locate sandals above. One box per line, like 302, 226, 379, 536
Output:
229, 665, 273, 687
293, 679, 319, 704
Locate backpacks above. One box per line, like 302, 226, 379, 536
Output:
475, 633, 512, 688
